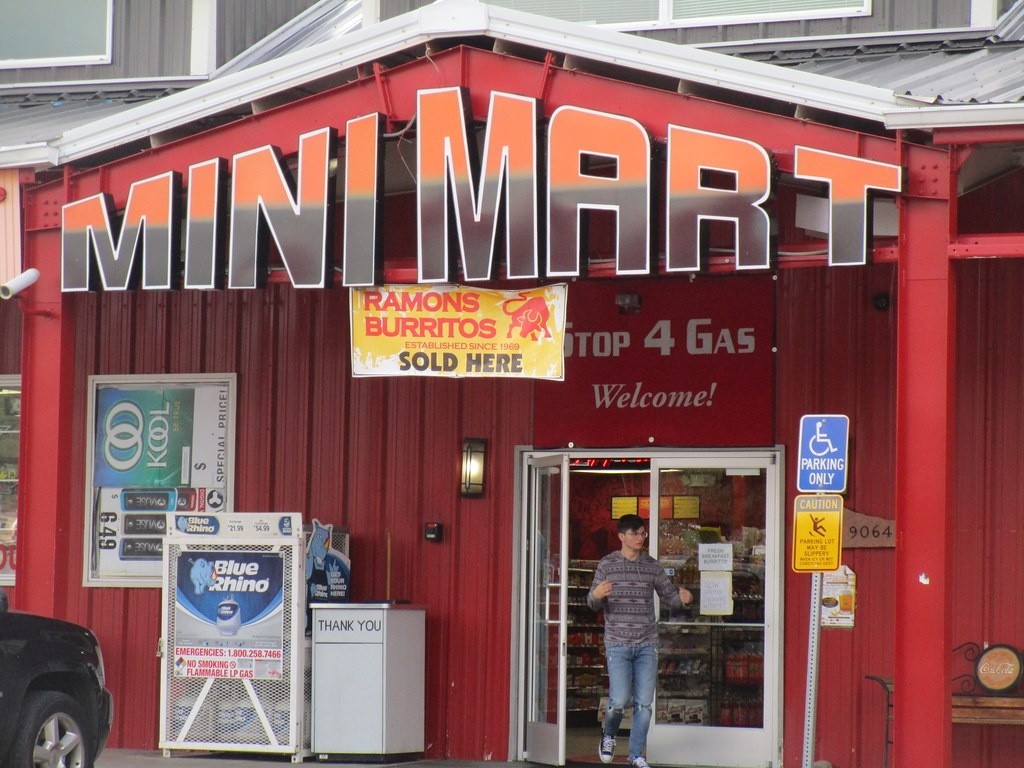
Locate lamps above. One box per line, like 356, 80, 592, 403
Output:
461, 437, 487, 497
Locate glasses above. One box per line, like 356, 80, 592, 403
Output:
623, 531, 648, 538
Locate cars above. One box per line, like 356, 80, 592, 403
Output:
0, 588, 115, 768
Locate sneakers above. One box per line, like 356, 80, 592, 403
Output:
598, 731, 616, 763
629, 756, 650, 768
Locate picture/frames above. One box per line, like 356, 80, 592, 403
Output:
611, 494, 701, 520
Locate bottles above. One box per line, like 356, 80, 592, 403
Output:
732, 591, 764, 623
719, 690, 763, 727
724, 650, 764, 687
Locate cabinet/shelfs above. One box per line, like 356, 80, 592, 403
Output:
0, 374, 22, 586
309, 603, 426, 763
539, 556, 766, 737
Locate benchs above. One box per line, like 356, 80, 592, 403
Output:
865, 642, 1024, 768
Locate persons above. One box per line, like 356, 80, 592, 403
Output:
587, 514, 695, 768
